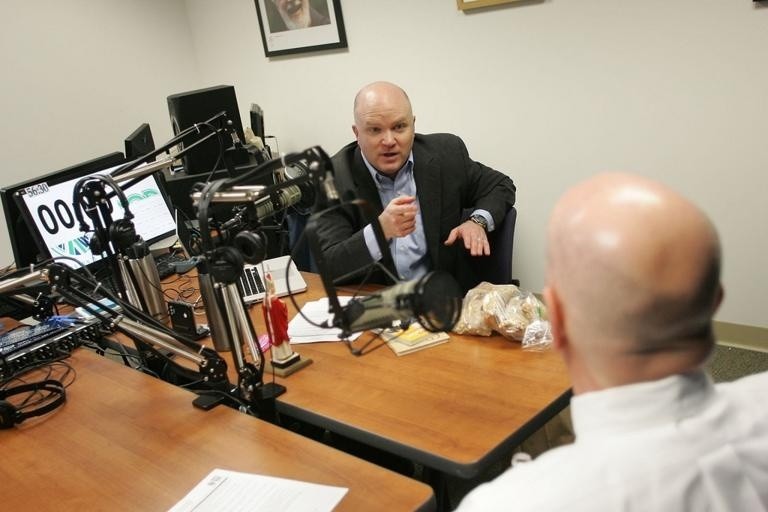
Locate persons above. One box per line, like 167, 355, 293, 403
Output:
268, 0, 329, 33
309, 81, 517, 304
450, 172, 768, 511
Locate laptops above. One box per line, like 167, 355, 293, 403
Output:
175, 209, 308, 305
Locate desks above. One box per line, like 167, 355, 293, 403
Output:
2, 313, 438, 512
44, 257, 577, 512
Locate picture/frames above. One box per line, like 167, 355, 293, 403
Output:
253, 0, 348, 58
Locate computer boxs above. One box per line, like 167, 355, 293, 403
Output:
159, 145, 271, 221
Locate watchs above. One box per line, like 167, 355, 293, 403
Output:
469, 210, 490, 231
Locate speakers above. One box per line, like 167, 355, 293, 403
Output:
166, 84, 250, 175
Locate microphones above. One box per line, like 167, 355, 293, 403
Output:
225, 182, 302, 225
334, 266, 428, 332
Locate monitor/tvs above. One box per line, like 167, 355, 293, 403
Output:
13, 150, 176, 276
1, 151, 127, 270
250, 103, 266, 147
124, 123, 155, 163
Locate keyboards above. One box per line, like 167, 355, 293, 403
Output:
63, 253, 188, 308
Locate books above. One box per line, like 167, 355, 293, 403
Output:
384, 328, 450, 358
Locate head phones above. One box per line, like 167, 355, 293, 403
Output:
70, 173, 136, 256
189, 178, 269, 286
0, 379, 67, 430
